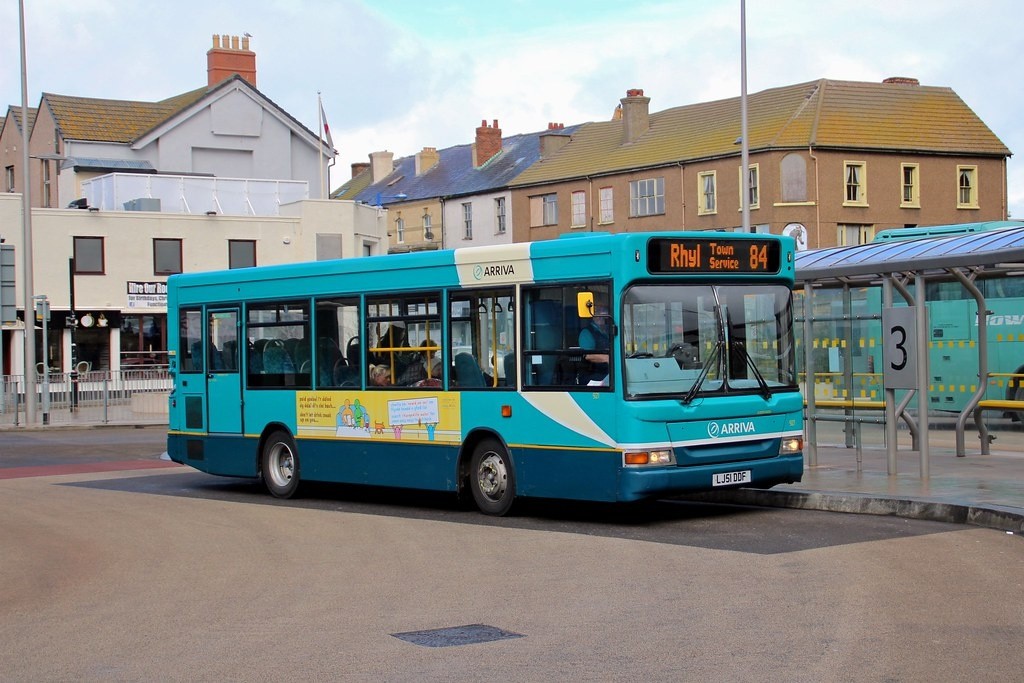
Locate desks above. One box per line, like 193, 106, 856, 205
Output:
49, 368, 62, 381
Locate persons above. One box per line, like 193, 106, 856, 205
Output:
370, 321, 446, 390
579, 303, 613, 380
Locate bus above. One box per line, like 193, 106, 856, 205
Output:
163, 227, 809, 516
866, 219, 1024, 430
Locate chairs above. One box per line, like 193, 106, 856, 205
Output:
190, 336, 515, 388
36, 361, 92, 382
553, 335, 578, 385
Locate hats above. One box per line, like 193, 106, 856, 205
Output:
392, 321, 405, 328
423, 357, 441, 373
